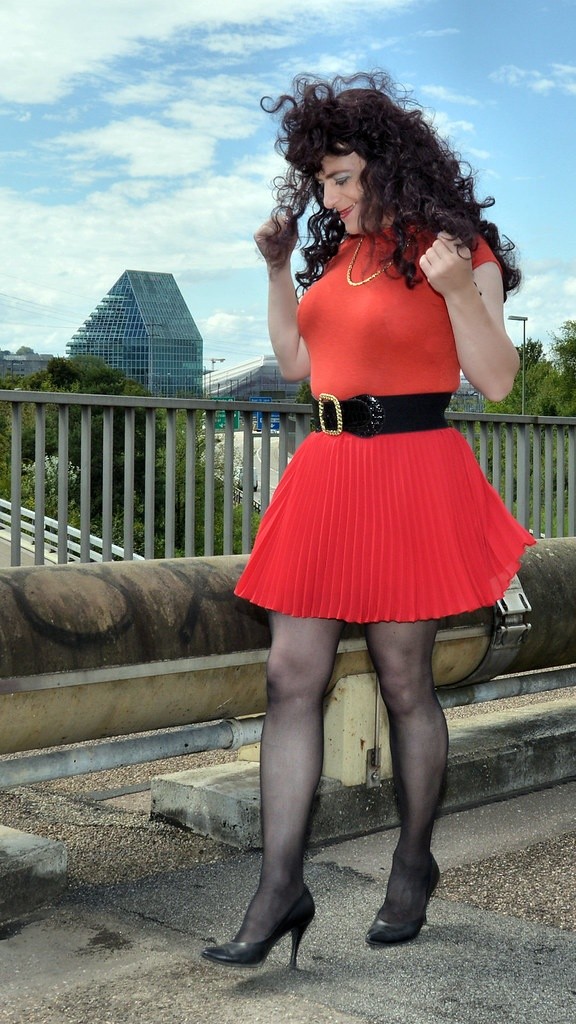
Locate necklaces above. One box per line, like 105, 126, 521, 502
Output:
347, 237, 410, 286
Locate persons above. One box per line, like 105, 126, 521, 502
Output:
201, 72, 536, 968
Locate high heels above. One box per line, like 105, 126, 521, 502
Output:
364, 853, 440, 945
201, 883, 315, 968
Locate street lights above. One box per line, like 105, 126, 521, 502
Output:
508, 315, 528, 415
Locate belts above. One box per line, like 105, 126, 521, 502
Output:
312, 392, 451, 437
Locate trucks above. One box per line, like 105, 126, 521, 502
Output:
231, 466, 258, 491
